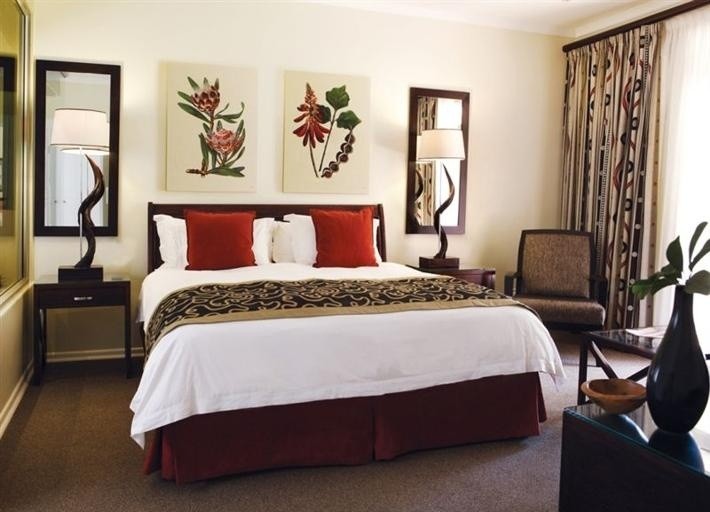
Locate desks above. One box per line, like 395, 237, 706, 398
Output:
551, 389, 710, 512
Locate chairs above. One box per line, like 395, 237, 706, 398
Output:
502, 225, 610, 370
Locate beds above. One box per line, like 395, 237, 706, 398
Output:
126, 196, 570, 488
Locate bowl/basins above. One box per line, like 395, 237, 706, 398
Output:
581, 378, 649, 415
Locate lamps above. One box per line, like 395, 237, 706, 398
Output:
417, 128, 465, 271
56, 119, 111, 201
416, 134, 441, 226
47, 106, 116, 284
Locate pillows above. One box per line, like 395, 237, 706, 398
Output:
150, 202, 389, 273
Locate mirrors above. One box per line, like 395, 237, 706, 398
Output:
401, 85, 472, 236
32, 56, 123, 239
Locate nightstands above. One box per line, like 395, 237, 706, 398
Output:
29, 270, 134, 382
403, 260, 497, 293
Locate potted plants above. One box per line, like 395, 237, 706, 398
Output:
624, 215, 710, 438
